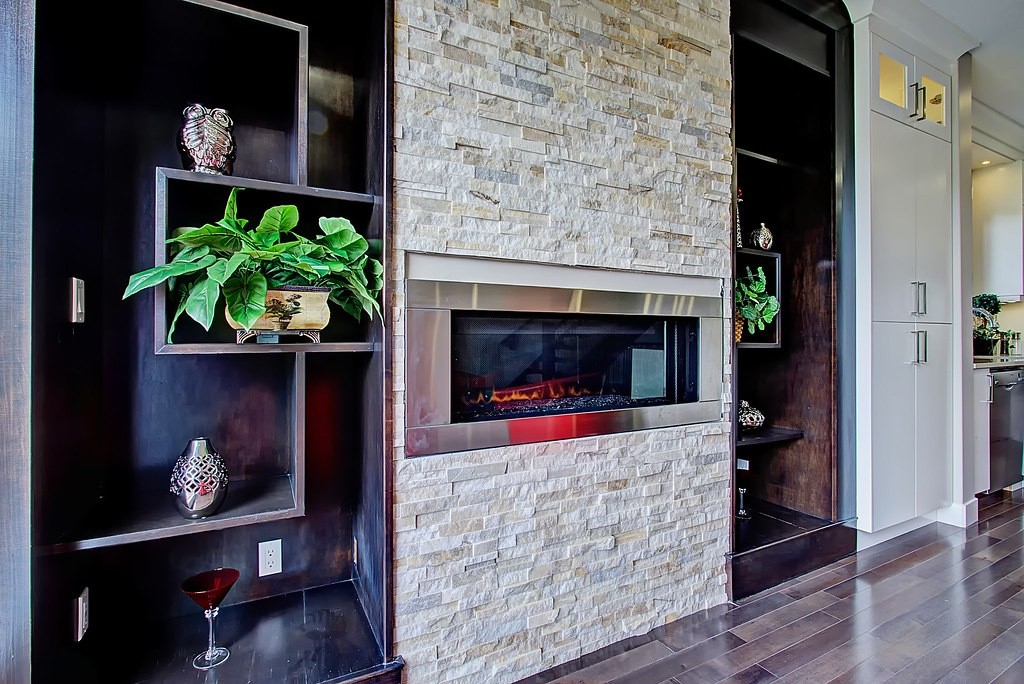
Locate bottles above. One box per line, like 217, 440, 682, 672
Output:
1000, 331, 1010, 356
1012, 331, 1021, 356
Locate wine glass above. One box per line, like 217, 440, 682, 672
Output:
179, 568, 241, 668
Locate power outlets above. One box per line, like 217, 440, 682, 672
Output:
257, 539, 282, 577
78, 587, 90, 640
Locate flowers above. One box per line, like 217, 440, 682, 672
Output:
736, 186, 742, 197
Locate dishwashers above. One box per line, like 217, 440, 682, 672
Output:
990, 369, 1024, 495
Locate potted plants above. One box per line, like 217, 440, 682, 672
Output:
972, 293, 1001, 356
734, 266, 780, 342
122, 188, 388, 345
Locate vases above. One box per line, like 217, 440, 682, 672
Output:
736, 198, 743, 248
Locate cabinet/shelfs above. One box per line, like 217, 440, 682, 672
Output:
854, 109, 954, 323
854, 16, 955, 143
974, 367, 990, 496
854, 323, 954, 552
0, 0, 405, 684
730, 0, 859, 602
